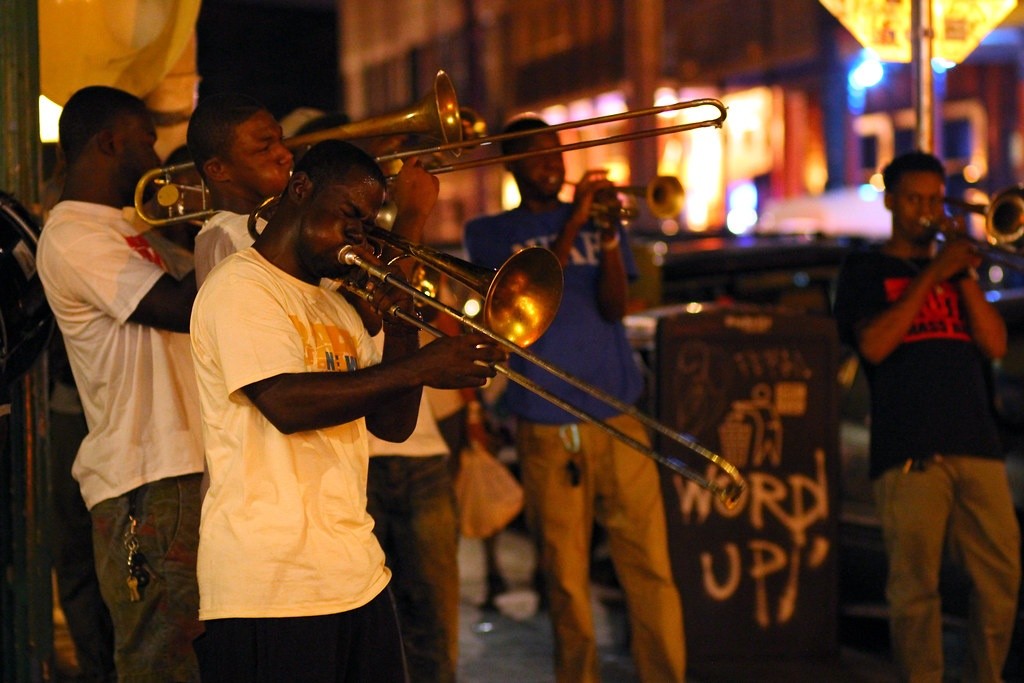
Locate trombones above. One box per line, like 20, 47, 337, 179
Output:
249, 195, 746, 509
919, 185, 1024, 270
133, 68, 728, 226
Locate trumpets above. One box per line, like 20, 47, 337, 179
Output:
547, 174, 686, 229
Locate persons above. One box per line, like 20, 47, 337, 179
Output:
190, 139, 508, 683
186, 93, 439, 301
36, 86, 218, 683
828, 151, 1022, 682
153, 142, 217, 254
466, 118, 687, 683
